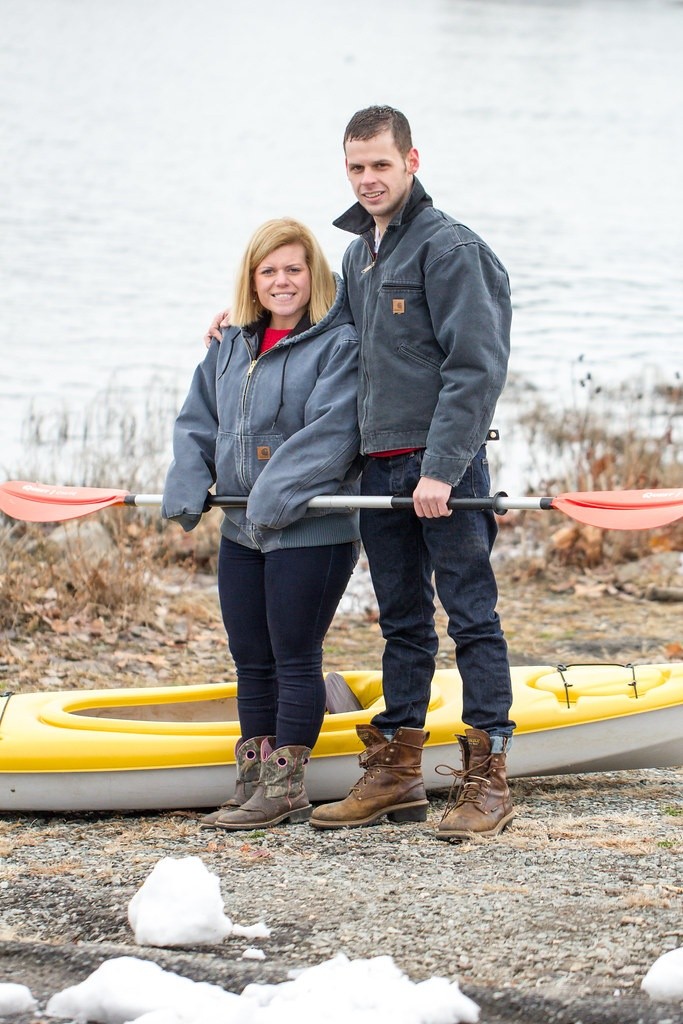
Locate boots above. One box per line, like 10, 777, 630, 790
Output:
201, 736, 271, 829
436, 728, 515, 841
215, 737, 312, 829
309, 724, 430, 829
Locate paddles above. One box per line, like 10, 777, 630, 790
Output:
0, 480, 683, 529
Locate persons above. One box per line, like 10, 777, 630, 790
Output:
208, 107, 512, 838
162, 220, 361, 830
